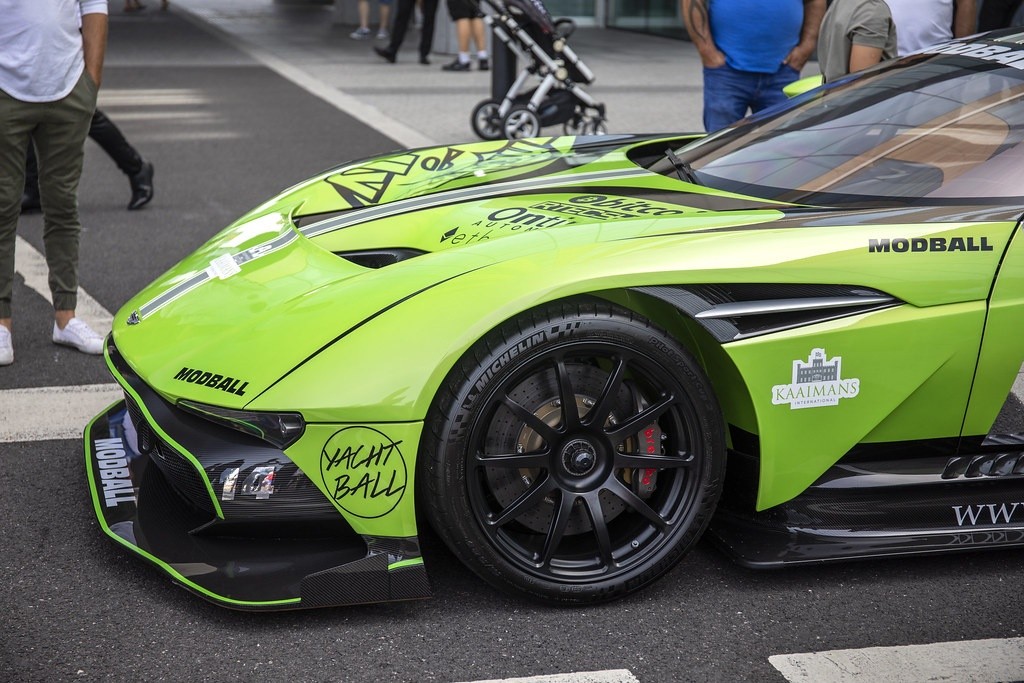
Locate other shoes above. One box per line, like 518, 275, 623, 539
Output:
377, 29, 390, 40
480, 58, 489, 70
374, 46, 395, 62
350, 26, 372, 39
443, 58, 469, 70
419, 55, 429, 64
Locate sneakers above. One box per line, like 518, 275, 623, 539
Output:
0, 326, 15, 366
52, 316, 105, 354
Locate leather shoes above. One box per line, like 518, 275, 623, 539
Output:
127, 161, 154, 210
19, 193, 41, 213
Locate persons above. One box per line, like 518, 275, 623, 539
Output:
123, 0, 492, 74
18, 105, 154, 217
815, 0, 1024, 85
0, 0, 110, 368
682, 0, 826, 136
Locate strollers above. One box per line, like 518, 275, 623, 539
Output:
471, 0, 608, 140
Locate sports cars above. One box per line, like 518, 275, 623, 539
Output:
83, 28, 1024, 611
